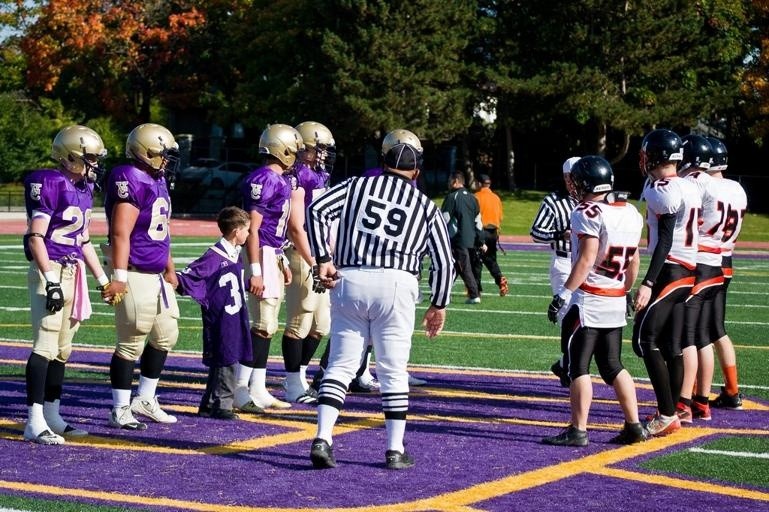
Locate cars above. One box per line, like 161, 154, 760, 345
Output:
204, 163, 252, 189
183, 158, 219, 179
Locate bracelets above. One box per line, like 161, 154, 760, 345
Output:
112, 268, 130, 283
251, 262, 263, 274
96, 273, 110, 287
560, 286, 572, 299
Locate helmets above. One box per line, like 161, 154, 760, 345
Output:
126, 124, 180, 182
51, 125, 107, 192
258, 121, 423, 174
562, 128, 729, 194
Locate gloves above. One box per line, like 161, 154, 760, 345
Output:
306, 265, 327, 294
47, 281, 64, 313
548, 291, 571, 324
97, 282, 129, 305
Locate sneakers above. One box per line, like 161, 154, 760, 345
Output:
542, 360, 743, 447
499, 276, 509, 296
465, 297, 481, 303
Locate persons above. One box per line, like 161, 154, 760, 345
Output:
286, 120, 338, 404
468, 172, 509, 298
541, 155, 648, 447
530, 156, 584, 387
232, 124, 305, 414
306, 128, 454, 469
174, 207, 266, 419
631, 129, 747, 438
101, 120, 181, 429
443, 171, 489, 304
22, 125, 128, 446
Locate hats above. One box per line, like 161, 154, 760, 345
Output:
477, 173, 492, 184
